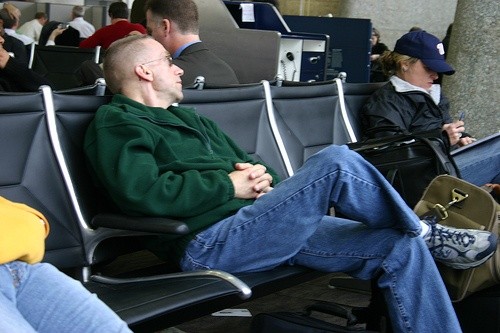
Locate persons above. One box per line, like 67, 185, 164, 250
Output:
83, 35, 498, 333
359, 27, 500, 210
0, 0, 256, 94
0, 197, 133, 333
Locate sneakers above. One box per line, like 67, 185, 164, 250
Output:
419, 219, 498, 271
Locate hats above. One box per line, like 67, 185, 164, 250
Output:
393, 30, 456, 76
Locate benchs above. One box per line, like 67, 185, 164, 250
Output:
25, 42, 101, 91
1, 71, 391, 333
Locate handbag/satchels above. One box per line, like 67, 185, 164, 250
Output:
336, 124, 464, 220
409, 174, 500, 302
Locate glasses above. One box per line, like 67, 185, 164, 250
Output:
138, 54, 174, 85
